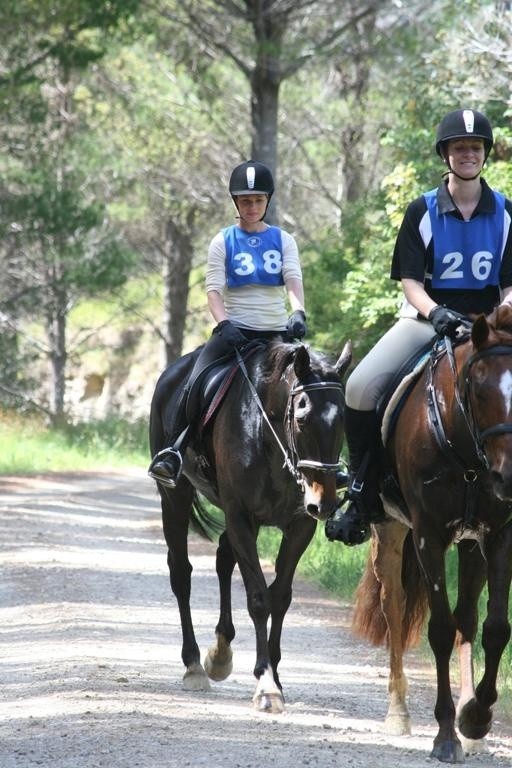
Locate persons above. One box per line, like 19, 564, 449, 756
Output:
150, 162, 352, 490
324, 108, 512, 548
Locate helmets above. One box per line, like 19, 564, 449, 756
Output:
229, 160, 274, 196
436, 109, 493, 160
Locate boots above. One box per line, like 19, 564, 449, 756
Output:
152, 388, 203, 481
325, 404, 385, 547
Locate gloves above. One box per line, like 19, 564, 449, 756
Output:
428, 305, 466, 340
218, 320, 249, 347
286, 310, 306, 338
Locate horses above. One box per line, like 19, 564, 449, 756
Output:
149, 338, 354, 715
348, 306, 512, 764
